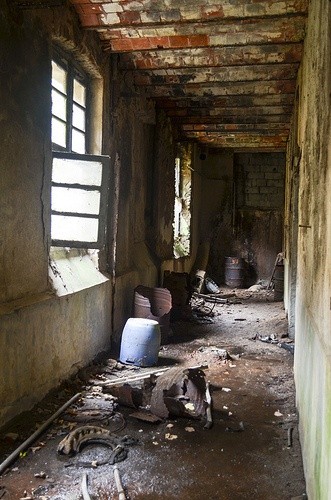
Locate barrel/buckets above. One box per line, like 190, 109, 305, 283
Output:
118, 317, 162, 367
274, 265, 284, 301
223, 255, 245, 287
